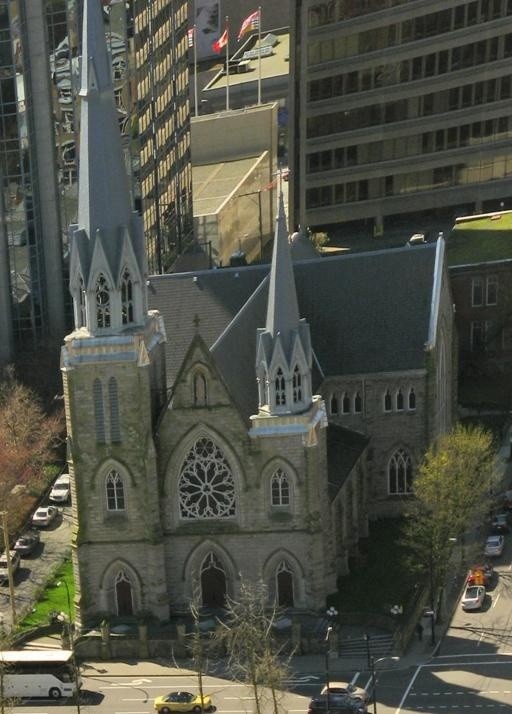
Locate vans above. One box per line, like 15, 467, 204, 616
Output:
0, 549, 20, 582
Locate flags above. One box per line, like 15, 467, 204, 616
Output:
189, 28, 193, 48
212, 29, 228, 55
237, 10, 259, 42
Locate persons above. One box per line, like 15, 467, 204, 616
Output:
416, 622, 425, 641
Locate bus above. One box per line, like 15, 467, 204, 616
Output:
0, 650, 80, 699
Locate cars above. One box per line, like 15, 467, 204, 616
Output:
493, 489, 512, 533
462, 584, 486, 610
321, 681, 369, 704
483, 535, 504, 558
467, 562, 493, 587
15, 532, 38, 556
154, 691, 212, 713
32, 505, 58, 527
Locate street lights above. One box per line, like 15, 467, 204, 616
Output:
391, 604, 404, 626
326, 607, 338, 630
371, 655, 400, 714
57, 578, 80, 713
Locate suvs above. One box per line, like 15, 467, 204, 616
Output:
308, 694, 367, 714
49, 474, 70, 503
